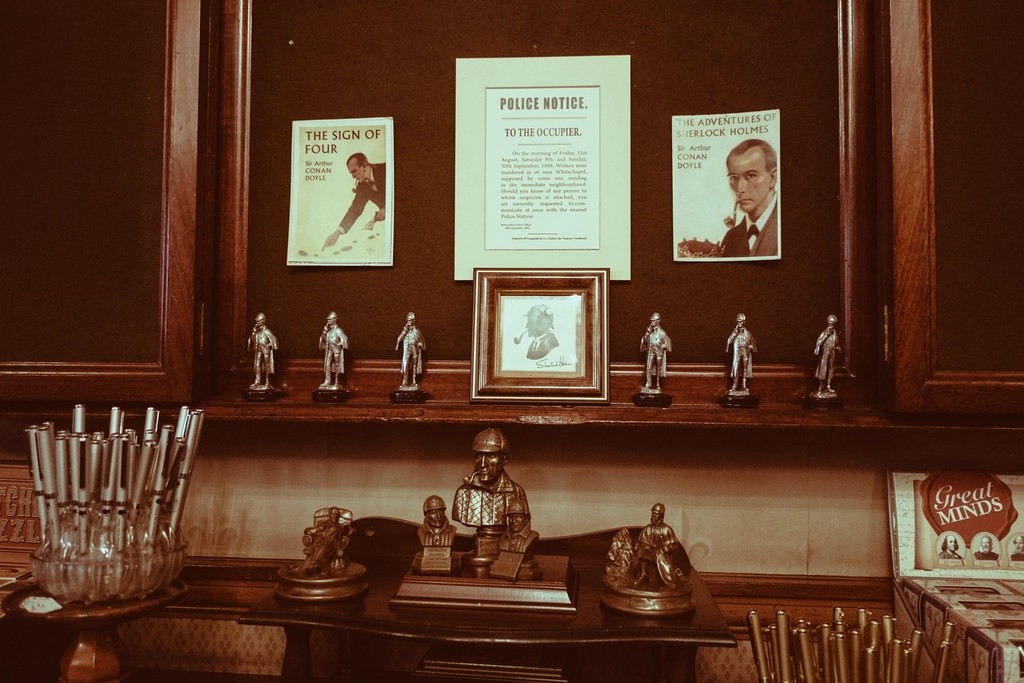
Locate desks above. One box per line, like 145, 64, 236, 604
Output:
237, 516, 738, 683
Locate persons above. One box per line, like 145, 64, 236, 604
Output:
395, 312, 426, 390
727, 313, 755, 393
637, 504, 678, 587
302, 507, 349, 567
639, 313, 672, 392
814, 314, 841, 393
318, 310, 349, 388
247, 313, 279, 385
452, 428, 531, 527
500, 500, 539, 557
416, 495, 457, 548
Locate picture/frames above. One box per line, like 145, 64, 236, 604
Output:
471, 267, 612, 401
887, 468, 1024, 581
